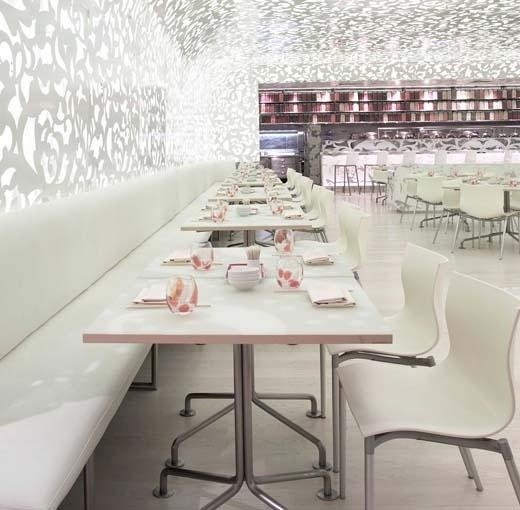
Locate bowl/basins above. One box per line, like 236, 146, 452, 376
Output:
226, 267, 260, 289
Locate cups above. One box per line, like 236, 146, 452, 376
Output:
166, 274, 197, 317
411, 165, 517, 187
274, 229, 296, 253
189, 240, 212, 270
275, 257, 304, 290
211, 171, 283, 225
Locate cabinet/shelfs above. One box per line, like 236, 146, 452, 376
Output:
257, 84, 519, 125
